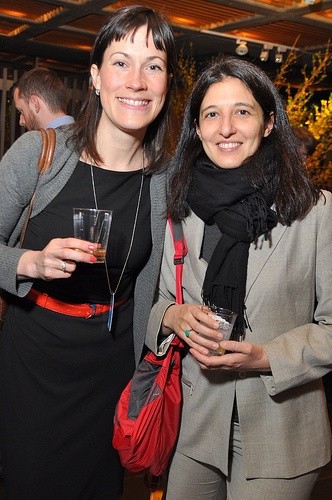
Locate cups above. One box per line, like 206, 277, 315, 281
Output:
200, 304, 235, 358
73, 207, 112, 263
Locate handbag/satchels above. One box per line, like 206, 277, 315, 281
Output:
111, 334, 186, 480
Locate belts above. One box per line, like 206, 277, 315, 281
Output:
24, 289, 129, 319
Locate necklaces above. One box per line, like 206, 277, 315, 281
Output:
91, 151, 144, 331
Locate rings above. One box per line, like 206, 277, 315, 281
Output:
62, 262, 66, 271
185, 329, 193, 336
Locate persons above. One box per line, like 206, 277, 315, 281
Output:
13, 67, 75, 130
0, 5, 177, 500
145, 58, 332, 500
